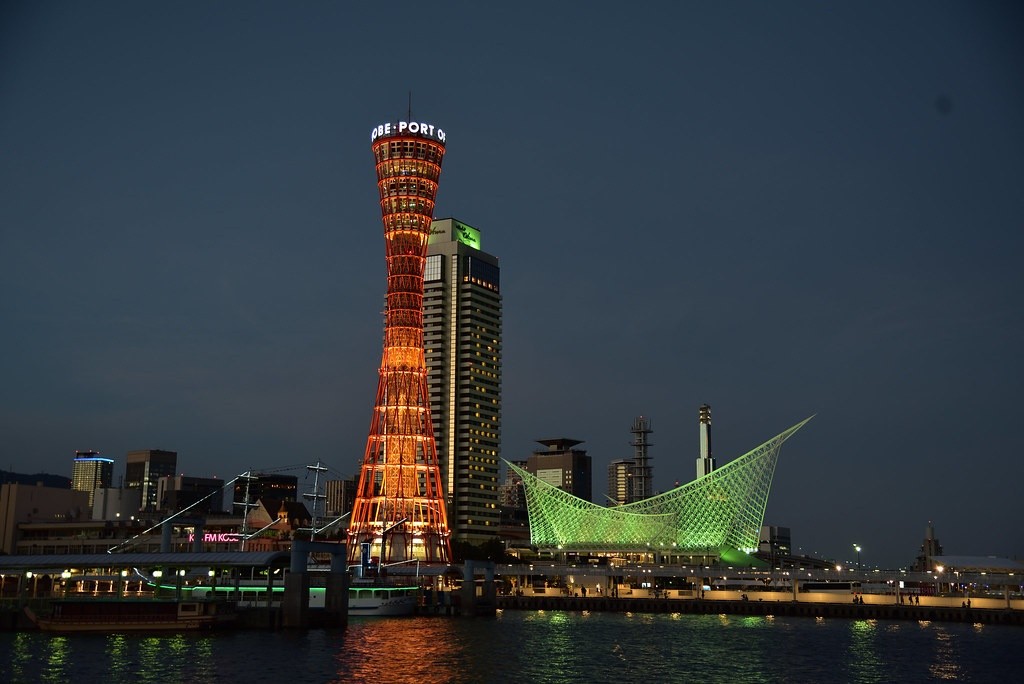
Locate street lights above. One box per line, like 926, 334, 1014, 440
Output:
920, 580, 922, 596
857, 547, 860, 570
791, 565, 796, 600
530, 567, 532, 589
783, 572, 789, 592
890, 581, 892, 595
935, 576, 937, 597
707, 547, 710, 565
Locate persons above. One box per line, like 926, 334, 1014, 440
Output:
513, 589, 523, 596
611, 587, 618, 597
699, 562, 704, 566
702, 590, 704, 598
581, 586, 586, 597
915, 595, 919, 606
855, 595, 864, 604
221, 577, 233, 585
653, 591, 659, 598
962, 601, 966, 608
967, 599, 970, 607
664, 591, 668, 599
206, 580, 210, 585
909, 595, 913, 605
741, 594, 748, 601
564, 584, 572, 597
901, 595, 904, 604
712, 559, 718, 566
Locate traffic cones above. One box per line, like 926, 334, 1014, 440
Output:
859, 592, 863, 600
854, 592, 856, 599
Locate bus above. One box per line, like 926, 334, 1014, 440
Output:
803, 581, 862, 593
451, 580, 506, 596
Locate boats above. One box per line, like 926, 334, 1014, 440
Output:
191, 586, 420, 615
23, 599, 237, 634
